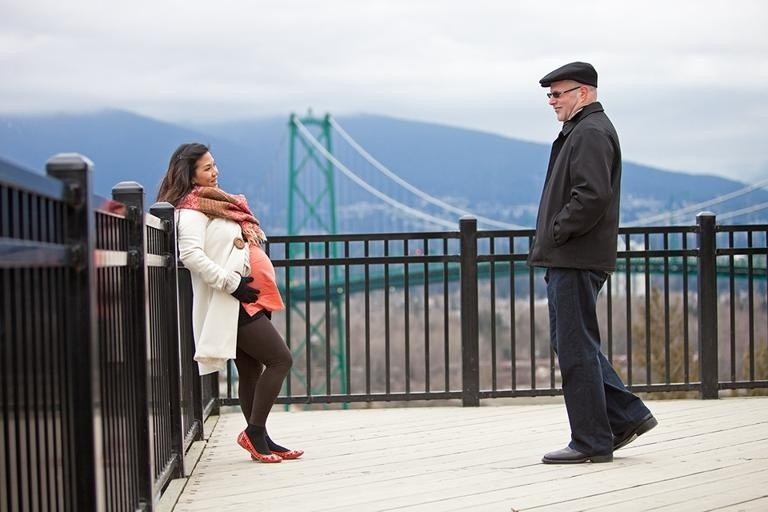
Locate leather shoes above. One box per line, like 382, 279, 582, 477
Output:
250, 447, 305, 461
612, 408, 659, 451
236, 429, 284, 464
541, 443, 615, 464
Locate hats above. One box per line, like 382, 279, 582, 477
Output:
537, 60, 600, 89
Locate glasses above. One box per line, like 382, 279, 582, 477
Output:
546, 86, 583, 98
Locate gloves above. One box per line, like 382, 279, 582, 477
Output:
228, 274, 261, 305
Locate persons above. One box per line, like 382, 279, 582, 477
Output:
155, 140, 307, 465
525, 59, 659, 467
